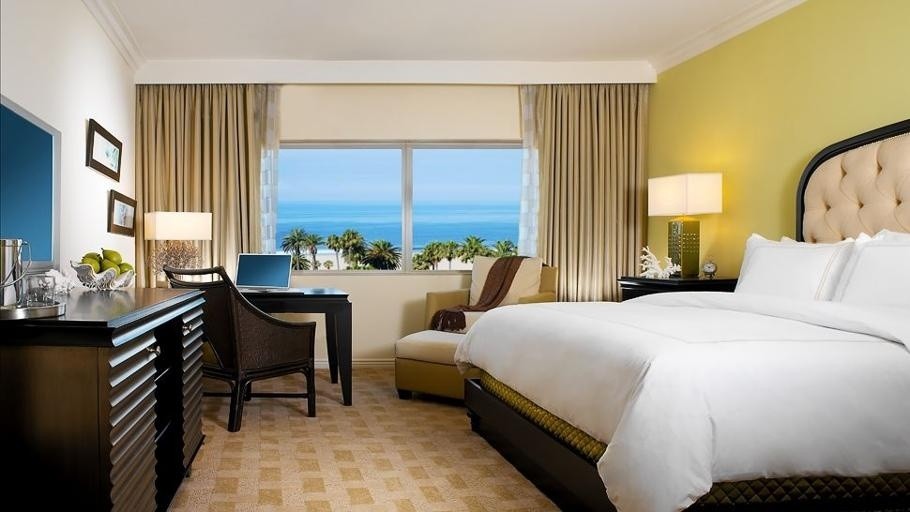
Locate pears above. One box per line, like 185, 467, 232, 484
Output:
82, 258, 101, 274
101, 247, 122, 265
99, 258, 120, 277
120, 262, 135, 275
84, 251, 102, 263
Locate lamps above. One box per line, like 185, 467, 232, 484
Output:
144, 212, 213, 278
647, 172, 723, 277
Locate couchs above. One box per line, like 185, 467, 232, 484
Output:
395, 266, 563, 400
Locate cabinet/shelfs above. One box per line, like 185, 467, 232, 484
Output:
1, 285, 204, 511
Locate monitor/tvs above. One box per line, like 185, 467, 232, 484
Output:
235, 252, 292, 293
2, 92, 62, 272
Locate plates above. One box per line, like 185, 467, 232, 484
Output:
71, 260, 135, 293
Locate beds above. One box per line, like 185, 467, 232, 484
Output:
454, 119, 910, 512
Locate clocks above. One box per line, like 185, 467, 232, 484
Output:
702, 261, 718, 278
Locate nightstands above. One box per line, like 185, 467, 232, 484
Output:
617, 274, 738, 301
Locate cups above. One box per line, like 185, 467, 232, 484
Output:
27, 274, 56, 307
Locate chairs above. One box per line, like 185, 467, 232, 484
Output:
163, 262, 318, 433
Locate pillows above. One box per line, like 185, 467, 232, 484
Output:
832, 241, 910, 307
733, 234, 854, 306
469, 254, 543, 310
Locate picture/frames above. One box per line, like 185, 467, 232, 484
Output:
86, 118, 123, 182
107, 189, 138, 237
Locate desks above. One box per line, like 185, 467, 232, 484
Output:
237, 284, 354, 406
